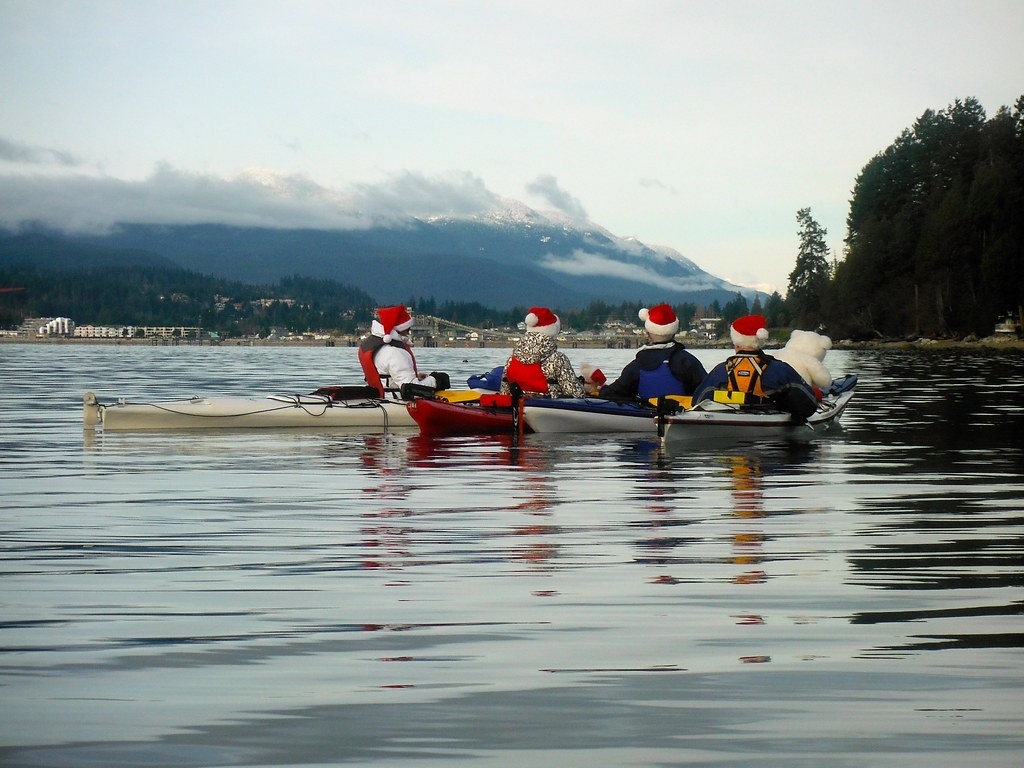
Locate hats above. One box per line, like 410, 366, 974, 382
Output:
729, 314, 769, 350
524, 307, 561, 338
638, 304, 680, 335
376, 306, 413, 344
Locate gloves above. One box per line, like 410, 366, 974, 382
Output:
429, 372, 451, 392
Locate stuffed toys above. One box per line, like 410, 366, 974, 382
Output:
579, 362, 607, 396
771, 329, 833, 393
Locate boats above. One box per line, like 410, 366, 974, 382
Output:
81, 384, 496, 431
400, 372, 863, 439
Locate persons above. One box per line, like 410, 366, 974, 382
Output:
357, 307, 451, 403
498, 307, 601, 399
598, 304, 708, 410
690, 314, 822, 419
